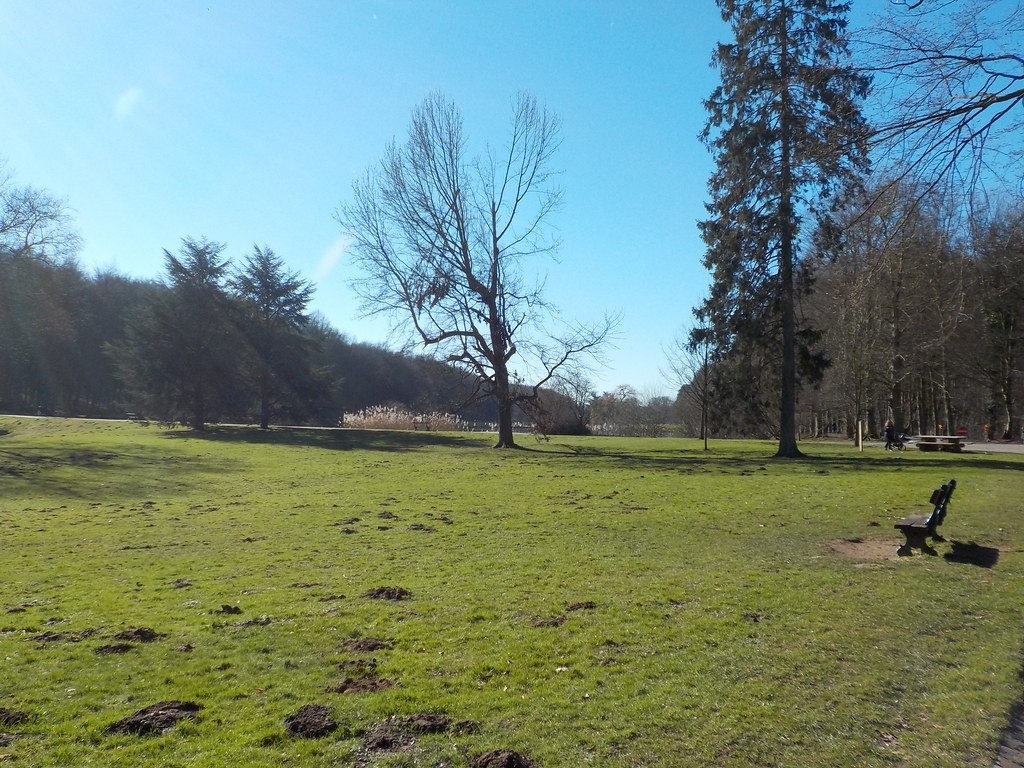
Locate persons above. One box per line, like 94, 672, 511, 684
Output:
884, 420, 895, 451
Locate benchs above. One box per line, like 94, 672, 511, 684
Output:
411, 417, 432, 431
894, 477, 956, 556
917, 442, 965, 447
890, 433, 906, 451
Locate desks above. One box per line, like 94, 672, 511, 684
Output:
914, 436, 967, 452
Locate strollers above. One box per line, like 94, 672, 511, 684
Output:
889, 433, 906, 451
